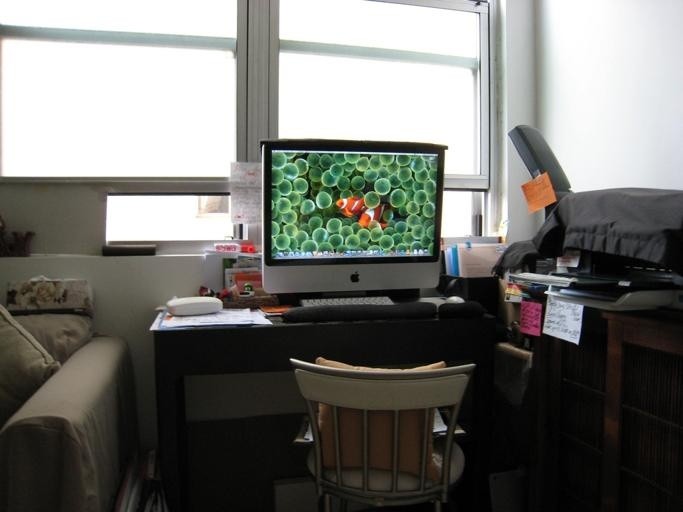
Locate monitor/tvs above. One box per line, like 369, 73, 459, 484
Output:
262, 139, 446, 293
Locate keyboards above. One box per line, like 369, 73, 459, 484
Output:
300, 295, 395, 308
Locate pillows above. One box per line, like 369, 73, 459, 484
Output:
314, 357, 447, 484
0, 301, 95, 401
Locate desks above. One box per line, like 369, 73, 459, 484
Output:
525, 299, 683, 512
149, 300, 498, 511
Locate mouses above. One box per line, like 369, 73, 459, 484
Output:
445, 295, 465, 303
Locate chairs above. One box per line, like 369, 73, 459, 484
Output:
288, 356, 478, 508
